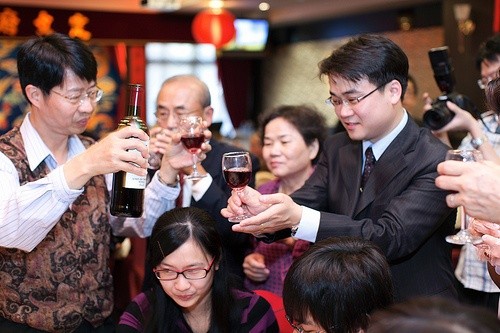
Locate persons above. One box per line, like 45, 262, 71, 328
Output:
424, 33, 500, 312
402, 75, 418, 113
367, 296, 500, 333
0, 32, 212, 333
117, 207, 282, 333
244, 104, 327, 297
146, 74, 262, 281
220, 34, 466, 305
282, 236, 394, 333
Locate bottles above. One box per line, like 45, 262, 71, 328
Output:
109, 83, 151, 219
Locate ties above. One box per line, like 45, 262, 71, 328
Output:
359, 145, 376, 191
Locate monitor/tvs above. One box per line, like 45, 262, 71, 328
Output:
221, 17, 272, 59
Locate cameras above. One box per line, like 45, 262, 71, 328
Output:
424, 46, 473, 130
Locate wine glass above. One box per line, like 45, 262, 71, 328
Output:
221, 151, 253, 223
444, 149, 482, 245
178, 115, 207, 180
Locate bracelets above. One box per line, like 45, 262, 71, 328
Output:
470, 135, 489, 149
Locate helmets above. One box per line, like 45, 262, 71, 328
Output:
154, 257, 216, 280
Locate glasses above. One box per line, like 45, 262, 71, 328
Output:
283, 311, 325, 333
52, 86, 105, 106
154, 109, 202, 121
326, 85, 383, 107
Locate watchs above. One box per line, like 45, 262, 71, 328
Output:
291, 224, 299, 236
